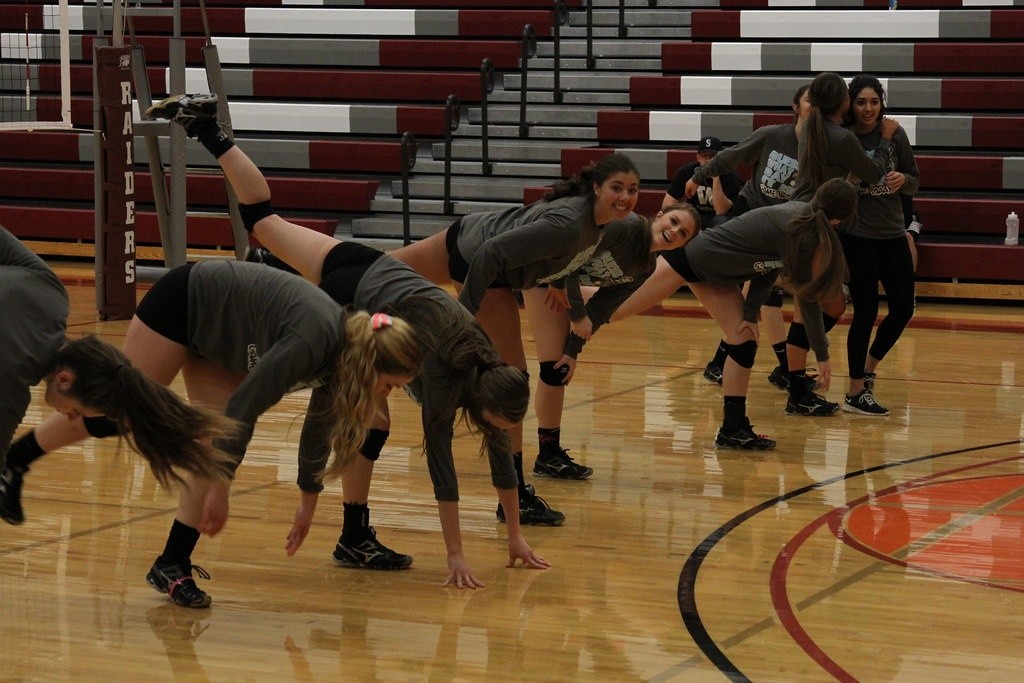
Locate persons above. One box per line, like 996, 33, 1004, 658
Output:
522, 201, 700, 482
662, 136, 741, 231
143, 92, 551, 589
0, 227, 250, 510
385, 157, 638, 527
577, 176, 856, 452
0, 258, 421, 609
678, 72, 920, 422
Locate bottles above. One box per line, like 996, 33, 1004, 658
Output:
1004, 212, 1019, 246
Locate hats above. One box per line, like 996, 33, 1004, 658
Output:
698, 135, 723, 153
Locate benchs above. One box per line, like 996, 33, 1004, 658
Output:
0, 0, 1024, 302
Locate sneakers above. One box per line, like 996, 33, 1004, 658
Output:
843, 390, 890, 416
0, 462, 30, 524
703, 362, 722, 386
146, 555, 211, 608
532, 449, 592, 479
785, 394, 839, 416
242, 246, 264, 264
332, 527, 413, 571
143, 94, 218, 138
715, 427, 776, 450
496, 485, 565, 526
767, 366, 815, 395
864, 371, 876, 395
